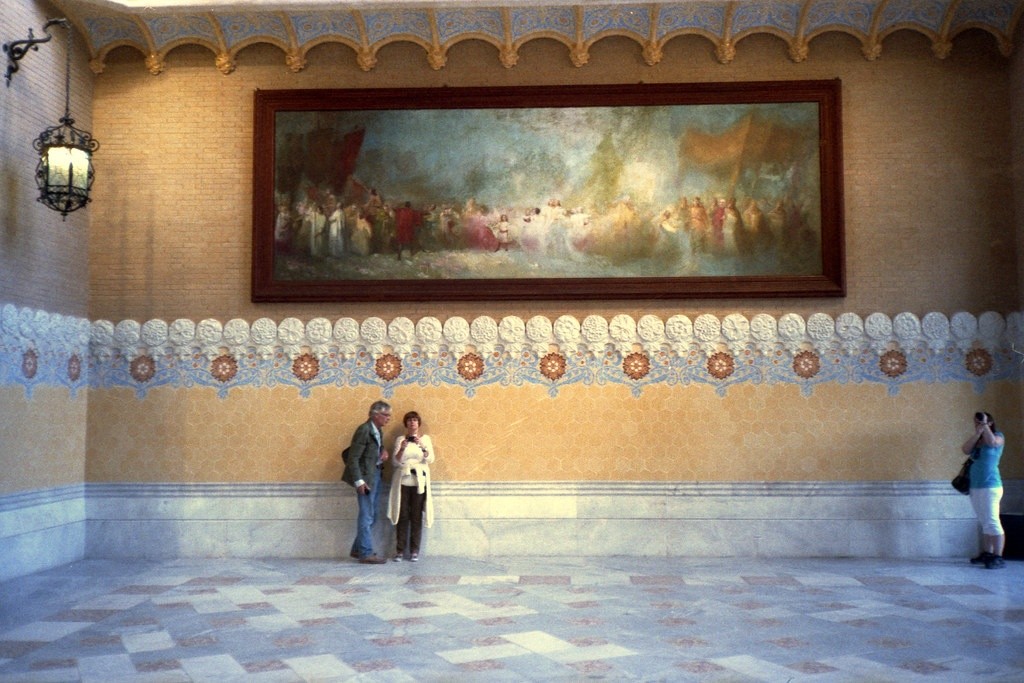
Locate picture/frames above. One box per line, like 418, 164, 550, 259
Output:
250, 77, 846, 303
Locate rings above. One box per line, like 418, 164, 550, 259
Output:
386, 456, 388, 457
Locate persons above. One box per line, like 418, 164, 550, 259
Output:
341, 400, 392, 564
962, 411, 1004, 569
387, 411, 435, 562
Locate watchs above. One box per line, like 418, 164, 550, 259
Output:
422, 446, 428, 452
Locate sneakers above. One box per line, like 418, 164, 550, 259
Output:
350, 551, 376, 559
970, 550, 993, 564
360, 556, 387, 564
985, 554, 1006, 568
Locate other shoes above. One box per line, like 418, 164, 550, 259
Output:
393, 554, 404, 562
410, 555, 419, 561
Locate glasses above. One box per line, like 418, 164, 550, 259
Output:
974, 448, 981, 459
381, 413, 391, 417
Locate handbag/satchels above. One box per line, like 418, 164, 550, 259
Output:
951, 459, 974, 495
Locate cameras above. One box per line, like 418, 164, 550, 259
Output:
406, 435, 416, 442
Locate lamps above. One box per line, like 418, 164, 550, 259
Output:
4, 16, 99, 222
